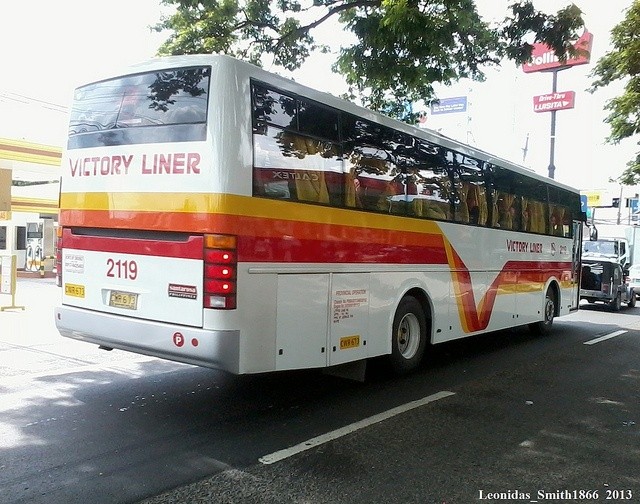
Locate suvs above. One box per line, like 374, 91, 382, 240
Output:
581, 236, 636, 311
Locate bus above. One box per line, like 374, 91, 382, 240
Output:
53, 52, 597, 393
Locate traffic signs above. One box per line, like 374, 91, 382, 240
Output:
430, 96, 467, 114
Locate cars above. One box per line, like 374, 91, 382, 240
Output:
631, 264, 640, 299
